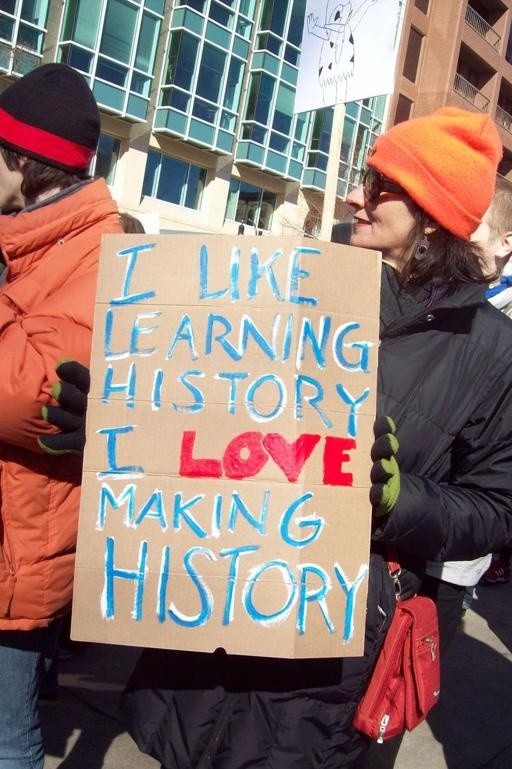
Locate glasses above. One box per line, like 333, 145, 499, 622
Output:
359, 166, 403, 203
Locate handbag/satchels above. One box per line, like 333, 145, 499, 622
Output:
350, 593, 443, 746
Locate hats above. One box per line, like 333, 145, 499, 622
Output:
365, 104, 505, 243
0, 60, 102, 175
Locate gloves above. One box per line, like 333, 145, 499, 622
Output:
369, 415, 401, 517
37, 357, 91, 458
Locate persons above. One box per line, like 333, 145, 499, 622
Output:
416, 175, 510, 646
34, 104, 511, 768
2, 60, 125, 768
